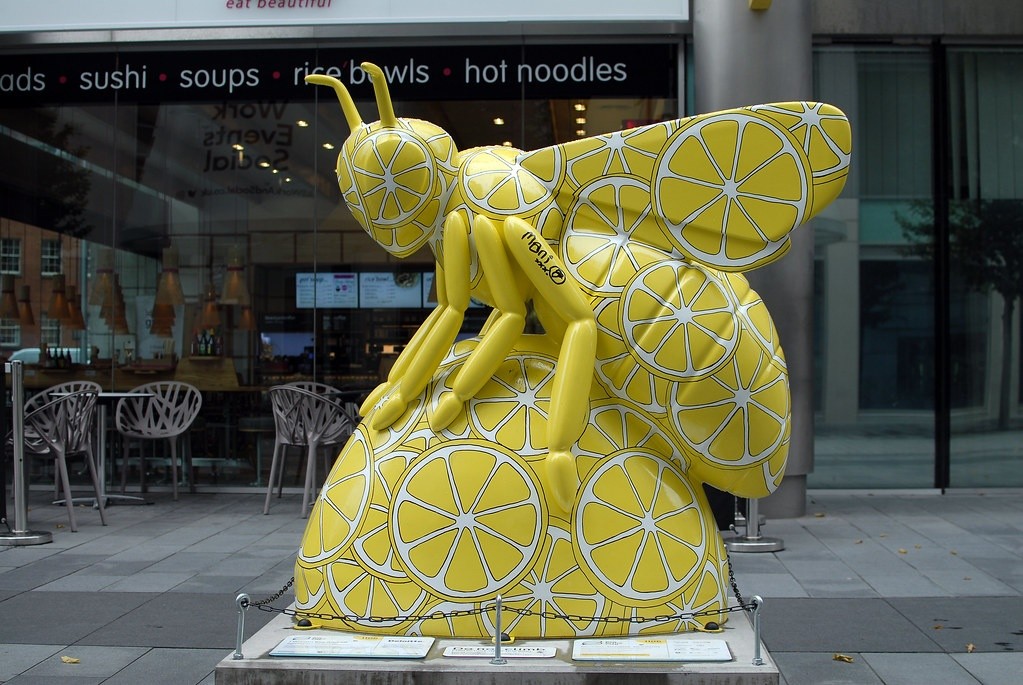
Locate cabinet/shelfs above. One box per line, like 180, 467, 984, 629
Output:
358, 309, 421, 358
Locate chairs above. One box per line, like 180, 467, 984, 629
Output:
116, 380, 203, 501
277, 382, 362, 507
5, 389, 110, 534
263, 385, 358, 519
10, 380, 103, 503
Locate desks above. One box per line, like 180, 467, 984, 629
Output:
47, 392, 156, 510
320, 389, 372, 397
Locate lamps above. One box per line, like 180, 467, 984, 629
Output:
0, 218, 21, 320
64, 237, 86, 330
17, 223, 36, 326
88, 107, 259, 339
46, 230, 71, 319
58, 234, 81, 325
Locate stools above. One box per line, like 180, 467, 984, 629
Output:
237, 416, 274, 488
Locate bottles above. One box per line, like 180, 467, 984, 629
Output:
190, 328, 223, 356
45, 345, 73, 368
